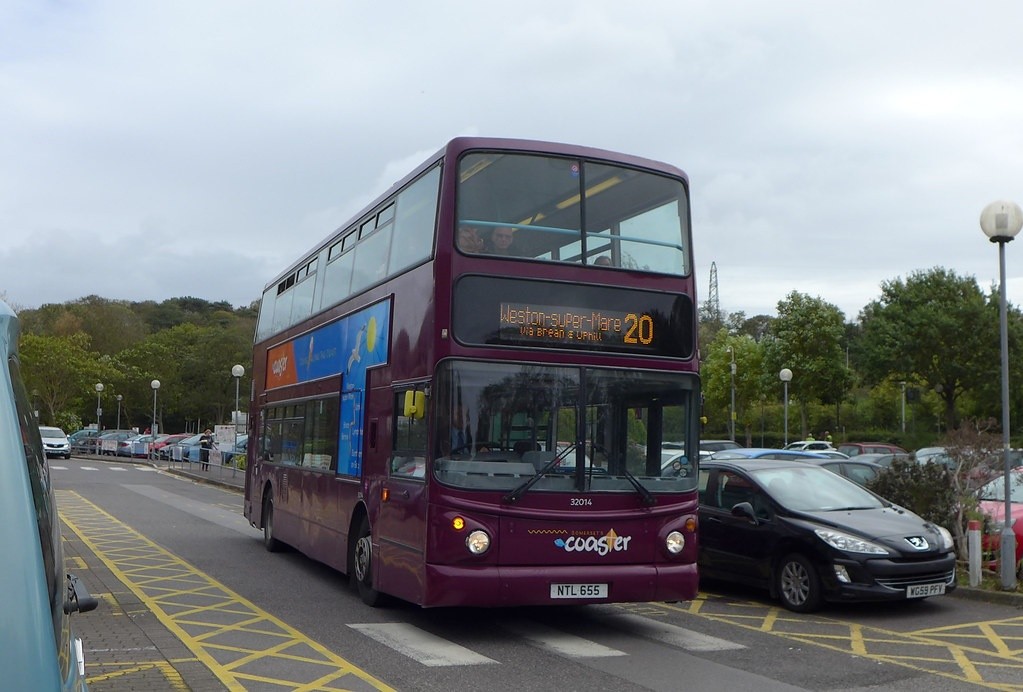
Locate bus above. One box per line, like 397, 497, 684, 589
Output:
243, 136, 703, 613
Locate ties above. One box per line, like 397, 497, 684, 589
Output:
457, 430, 464, 454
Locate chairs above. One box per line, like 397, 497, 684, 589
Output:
725, 476, 755, 510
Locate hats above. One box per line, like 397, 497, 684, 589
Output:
205, 429, 212, 434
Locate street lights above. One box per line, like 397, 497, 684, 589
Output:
979, 198, 1023, 591
116, 394, 123, 431
900, 382, 907, 431
726, 346, 736, 441
151, 379, 160, 459
232, 365, 244, 475
96, 383, 104, 456
780, 369, 794, 446
32, 389, 38, 409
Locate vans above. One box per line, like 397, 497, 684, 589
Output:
0, 300, 100, 692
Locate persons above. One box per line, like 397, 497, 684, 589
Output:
824, 431, 832, 442
806, 433, 815, 442
594, 256, 612, 266
449, 402, 491, 454
199, 429, 218, 471
458, 226, 525, 257
144, 427, 150, 434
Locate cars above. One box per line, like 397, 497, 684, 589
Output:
538, 439, 1023, 493
67, 428, 248, 463
38, 426, 71, 459
950, 463, 1023, 579
661, 464, 959, 614
